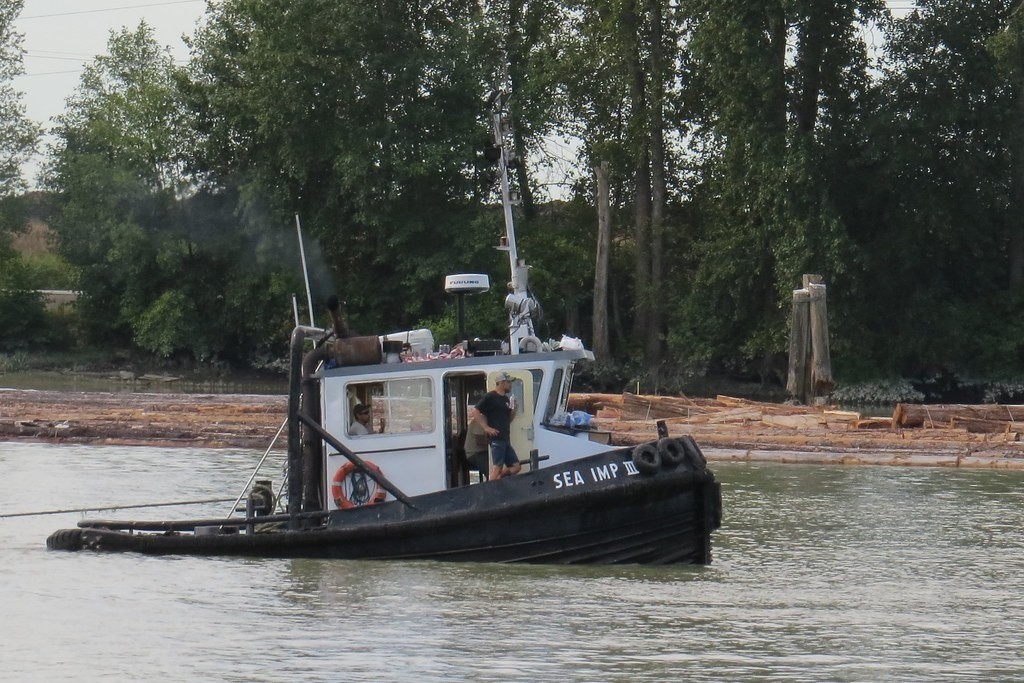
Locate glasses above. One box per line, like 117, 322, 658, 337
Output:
359, 410, 369, 415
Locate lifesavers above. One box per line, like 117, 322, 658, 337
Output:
632, 443, 662, 473
657, 437, 686, 465
680, 434, 709, 466
332, 459, 388, 508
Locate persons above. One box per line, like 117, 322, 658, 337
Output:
472, 372, 521, 481
464, 415, 489, 477
350, 404, 386, 434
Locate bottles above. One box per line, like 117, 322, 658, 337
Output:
509, 393, 516, 411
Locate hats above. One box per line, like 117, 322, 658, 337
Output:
495, 372, 516, 382
353, 404, 371, 414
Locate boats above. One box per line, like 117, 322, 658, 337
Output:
44, 112, 721, 567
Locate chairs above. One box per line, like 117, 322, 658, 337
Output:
455, 428, 489, 484
